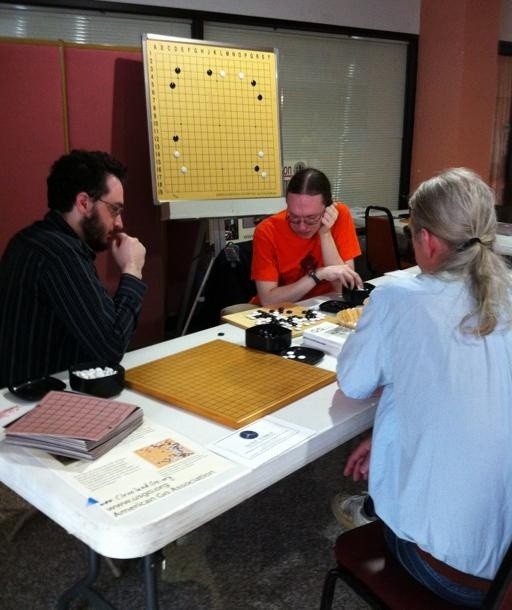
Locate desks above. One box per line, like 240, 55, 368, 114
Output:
225, 207, 408, 249
389, 202, 512, 257
2, 261, 498, 608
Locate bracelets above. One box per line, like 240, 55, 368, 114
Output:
308, 271, 320, 284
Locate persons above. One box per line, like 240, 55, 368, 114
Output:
0, 150, 147, 386
328, 162, 512, 610
247, 167, 364, 306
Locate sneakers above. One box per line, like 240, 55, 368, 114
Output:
331, 491, 378, 530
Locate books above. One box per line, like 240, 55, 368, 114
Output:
302, 320, 353, 357
5, 391, 144, 462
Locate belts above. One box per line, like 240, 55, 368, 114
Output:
418, 550, 492, 592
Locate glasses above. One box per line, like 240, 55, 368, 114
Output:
100, 199, 123, 217
285, 208, 325, 225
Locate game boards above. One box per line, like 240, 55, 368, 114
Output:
221, 302, 335, 338
123, 339, 337, 429
141, 33, 285, 205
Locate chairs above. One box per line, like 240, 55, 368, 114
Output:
363, 202, 402, 279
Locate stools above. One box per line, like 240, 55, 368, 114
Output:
317, 515, 512, 607
